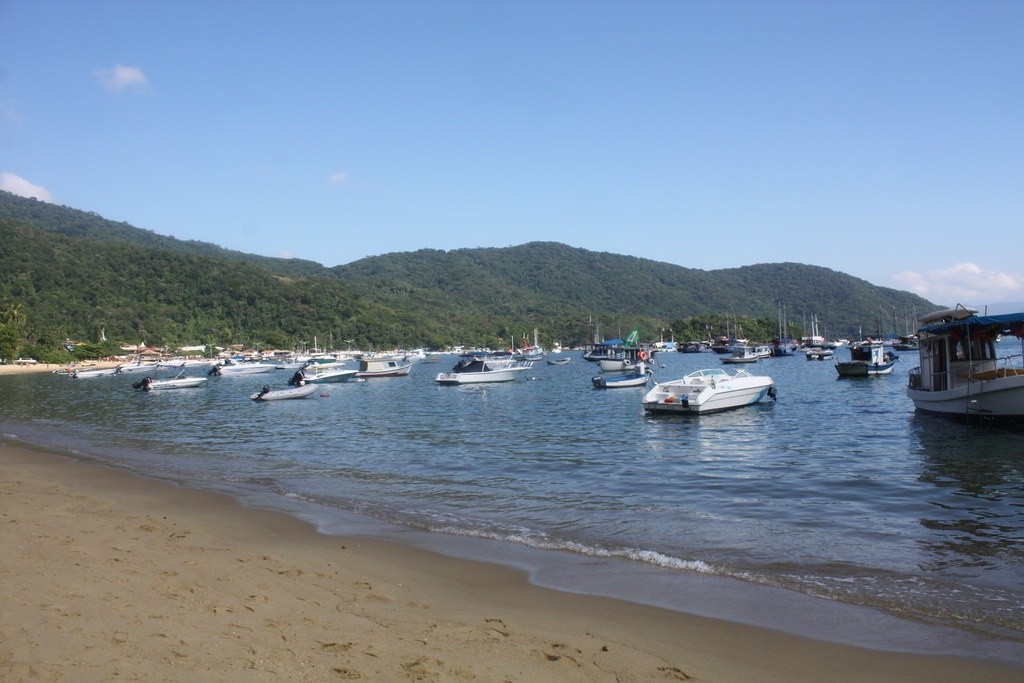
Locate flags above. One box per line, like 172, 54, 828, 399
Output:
624, 328, 639, 350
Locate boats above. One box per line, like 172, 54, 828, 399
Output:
891, 334, 920, 351
156, 347, 428, 368
641, 366, 778, 416
52, 367, 90, 375
435, 351, 534, 385
214, 358, 277, 376
433, 333, 546, 360
73, 362, 158, 379
580, 325, 655, 364
143, 376, 208, 390
834, 326, 899, 376
249, 383, 320, 401
598, 351, 646, 371
906, 303, 1024, 425
355, 357, 414, 379
547, 356, 571, 365
592, 361, 653, 389
287, 359, 358, 385
652, 300, 856, 363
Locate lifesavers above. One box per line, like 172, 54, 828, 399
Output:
68, 372, 74, 377
319, 393, 330, 397
636, 350, 647, 360
